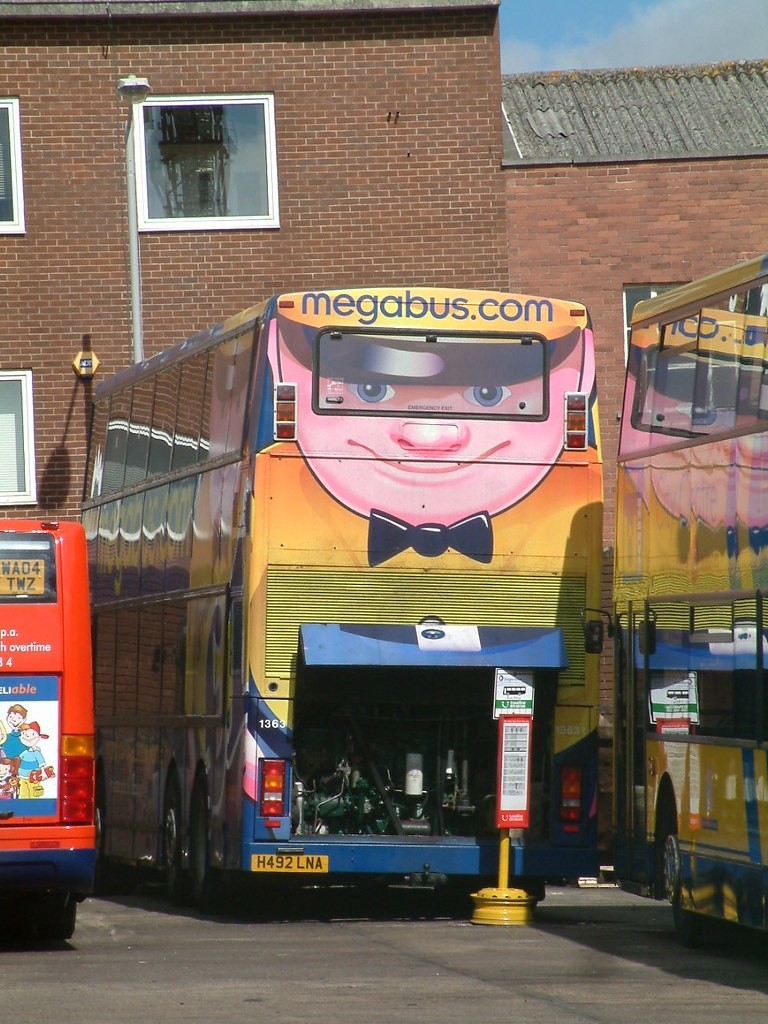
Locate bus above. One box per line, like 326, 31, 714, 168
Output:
0, 517, 97, 946
579, 250, 767, 943
73, 286, 604, 913
